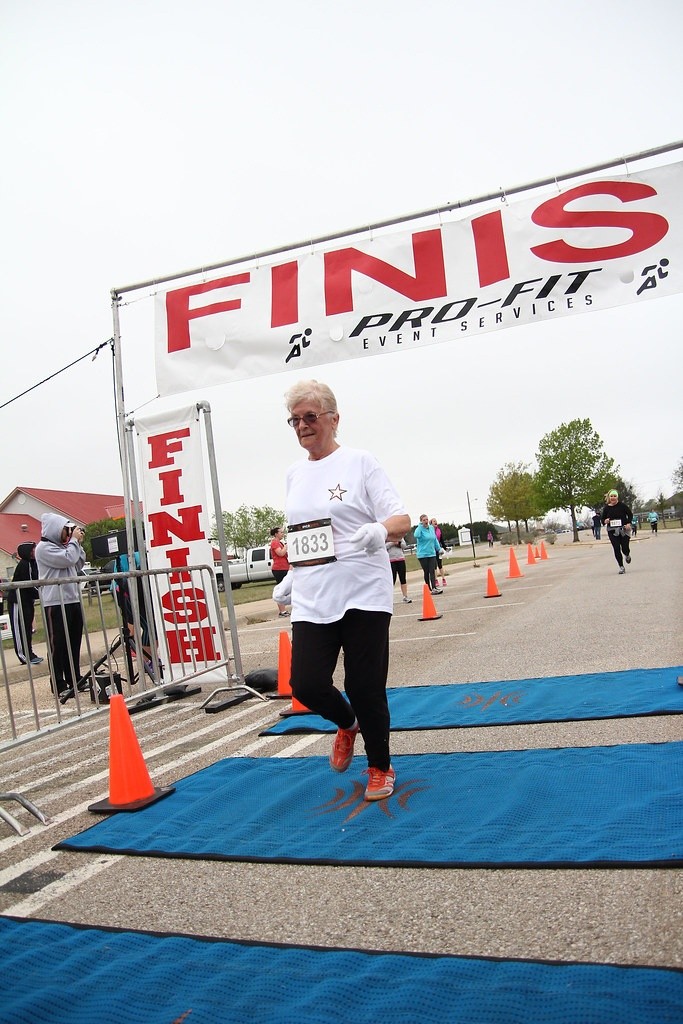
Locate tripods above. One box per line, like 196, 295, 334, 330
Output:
60, 526, 163, 705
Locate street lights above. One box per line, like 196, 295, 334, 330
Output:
467, 498, 479, 558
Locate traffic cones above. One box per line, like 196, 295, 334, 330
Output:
87, 693, 175, 812
483, 568, 503, 598
279, 694, 317, 716
417, 584, 443, 620
266, 631, 292, 699
539, 541, 550, 560
534, 546, 540, 558
525, 543, 539, 565
506, 547, 524, 579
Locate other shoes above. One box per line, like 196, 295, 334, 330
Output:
279, 610, 291, 617
619, 565, 626, 574
625, 554, 632, 564
59, 689, 74, 698
78, 682, 90, 691
29, 657, 42, 664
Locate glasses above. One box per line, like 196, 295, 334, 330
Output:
288, 411, 335, 427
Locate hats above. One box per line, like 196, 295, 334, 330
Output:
65, 522, 75, 527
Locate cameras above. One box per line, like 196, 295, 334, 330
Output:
71, 525, 85, 534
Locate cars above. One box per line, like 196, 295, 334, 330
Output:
546, 529, 571, 534
403, 544, 417, 553
76, 560, 116, 596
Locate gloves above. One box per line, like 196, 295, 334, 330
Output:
273, 569, 292, 603
349, 521, 389, 555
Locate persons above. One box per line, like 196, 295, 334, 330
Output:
429, 518, 449, 586
413, 514, 444, 594
648, 508, 658, 536
487, 531, 493, 547
601, 489, 633, 574
270, 527, 289, 616
272, 380, 411, 800
7, 541, 44, 665
590, 513, 601, 539
385, 537, 412, 604
109, 551, 147, 661
631, 515, 637, 537
35, 513, 90, 698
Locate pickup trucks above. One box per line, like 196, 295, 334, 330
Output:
214, 546, 276, 593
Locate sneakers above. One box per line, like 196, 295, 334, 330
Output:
432, 588, 442, 594
330, 722, 360, 773
402, 597, 412, 603
442, 578, 446, 586
435, 580, 440, 587
364, 765, 395, 801
